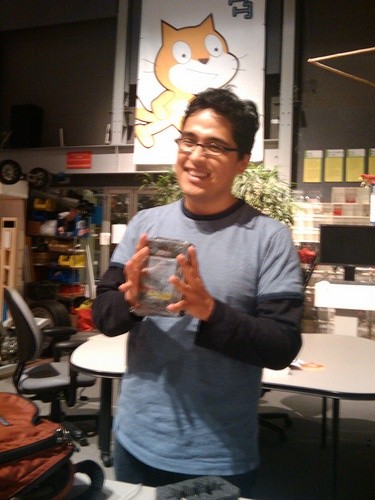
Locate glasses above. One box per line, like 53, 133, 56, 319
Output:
174, 138, 240, 156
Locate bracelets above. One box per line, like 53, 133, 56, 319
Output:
130, 307, 145, 316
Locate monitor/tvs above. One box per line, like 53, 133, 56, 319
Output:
318, 225, 375, 284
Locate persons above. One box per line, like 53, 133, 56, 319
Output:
91, 88, 304, 500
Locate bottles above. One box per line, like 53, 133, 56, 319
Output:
359, 174, 375, 203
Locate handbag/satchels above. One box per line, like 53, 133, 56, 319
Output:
71, 296, 94, 332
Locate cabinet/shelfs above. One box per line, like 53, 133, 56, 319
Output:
96, 187, 163, 283
26, 190, 95, 289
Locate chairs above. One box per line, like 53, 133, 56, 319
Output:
4, 287, 96, 427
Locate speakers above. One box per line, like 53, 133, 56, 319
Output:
8, 103, 44, 148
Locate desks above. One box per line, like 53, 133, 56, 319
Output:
73, 318, 375, 500
